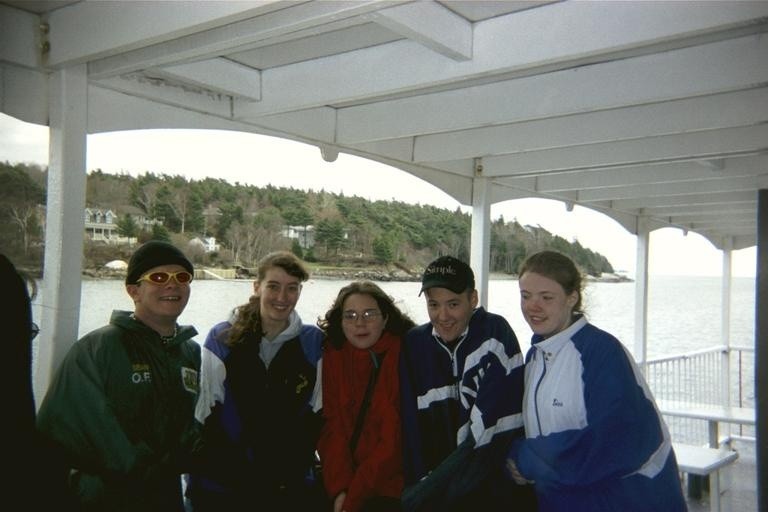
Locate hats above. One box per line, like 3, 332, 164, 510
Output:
418, 256, 475, 297
126, 240, 194, 286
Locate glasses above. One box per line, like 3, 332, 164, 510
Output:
135, 271, 193, 286
342, 309, 390, 322
